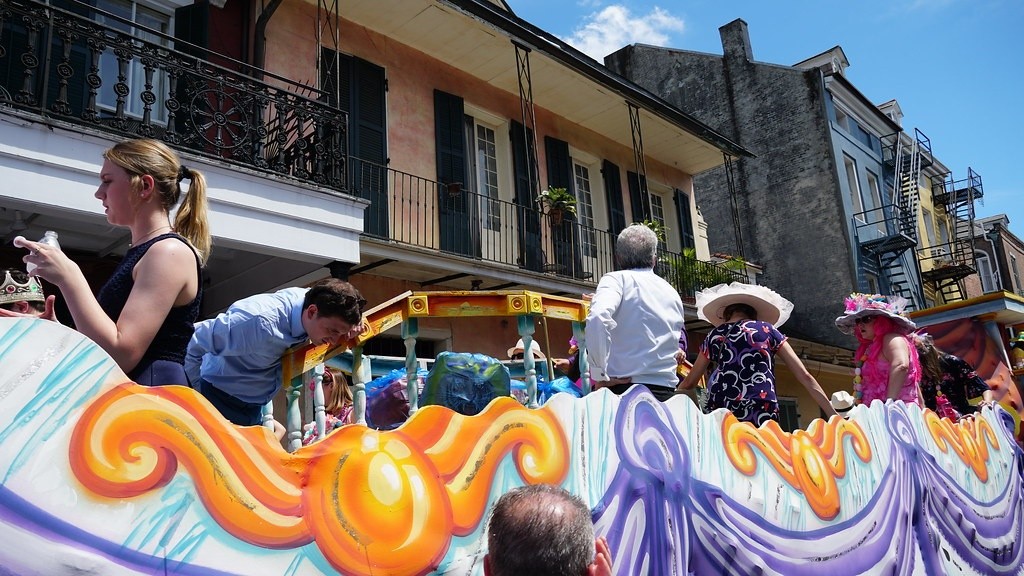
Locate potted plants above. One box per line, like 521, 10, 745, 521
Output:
535, 185, 577, 225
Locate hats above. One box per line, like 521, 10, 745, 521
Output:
831, 390, 857, 419
907, 327, 928, 342
506, 338, 546, 360
694, 281, 794, 329
835, 293, 917, 335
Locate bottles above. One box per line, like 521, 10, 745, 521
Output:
26, 230, 62, 272
936, 395, 956, 424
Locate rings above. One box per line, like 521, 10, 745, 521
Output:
34, 248, 40, 255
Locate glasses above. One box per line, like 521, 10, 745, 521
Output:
323, 379, 332, 387
854, 316, 878, 324
324, 291, 370, 311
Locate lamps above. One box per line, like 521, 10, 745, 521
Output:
832, 356, 839, 364
801, 349, 807, 359
10, 210, 28, 230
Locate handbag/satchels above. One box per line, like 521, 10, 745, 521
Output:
936, 394, 962, 422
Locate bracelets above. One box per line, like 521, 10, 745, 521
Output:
853, 354, 868, 405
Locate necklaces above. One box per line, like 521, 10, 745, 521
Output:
128, 226, 168, 248
338, 404, 347, 420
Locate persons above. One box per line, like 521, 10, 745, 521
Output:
916, 333, 992, 423
830, 391, 856, 420
310, 367, 356, 424
0, 139, 212, 386
484, 483, 613, 576
677, 281, 840, 428
508, 338, 546, 360
0, 268, 45, 315
185, 278, 367, 443
835, 293, 923, 410
554, 224, 687, 402
